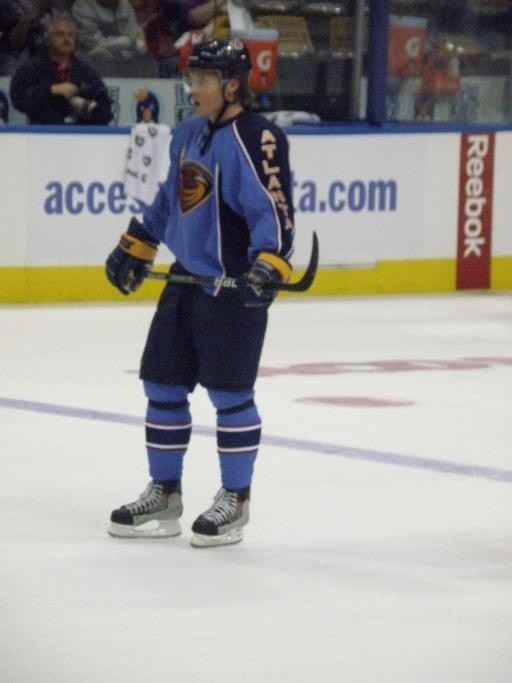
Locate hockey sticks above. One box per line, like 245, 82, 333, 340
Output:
119, 229, 319, 291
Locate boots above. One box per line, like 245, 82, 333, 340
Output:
111, 480, 183, 524
192, 486, 249, 535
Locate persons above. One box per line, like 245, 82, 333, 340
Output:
104, 34, 294, 538
0, 0, 270, 130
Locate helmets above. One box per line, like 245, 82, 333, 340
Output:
182, 36, 251, 93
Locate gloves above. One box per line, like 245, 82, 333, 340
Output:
233, 249, 293, 303
105, 216, 160, 295
